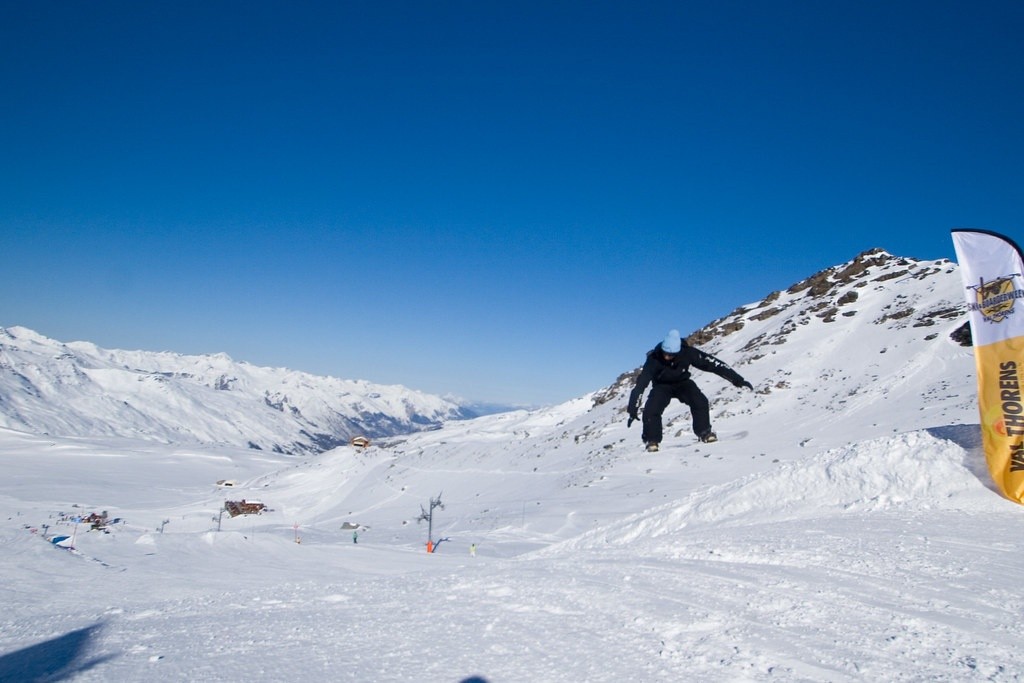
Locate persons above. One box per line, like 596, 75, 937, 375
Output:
297, 537, 300, 544
353, 532, 357, 543
627, 330, 753, 452
470, 544, 476, 557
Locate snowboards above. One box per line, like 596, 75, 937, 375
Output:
620, 429, 749, 456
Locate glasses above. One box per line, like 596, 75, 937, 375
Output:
663, 351, 675, 356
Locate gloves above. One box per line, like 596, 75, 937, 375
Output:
628, 412, 640, 428
737, 380, 753, 391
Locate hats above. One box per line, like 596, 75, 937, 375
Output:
660, 329, 681, 353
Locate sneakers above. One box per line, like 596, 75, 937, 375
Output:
701, 433, 717, 443
645, 441, 658, 452
